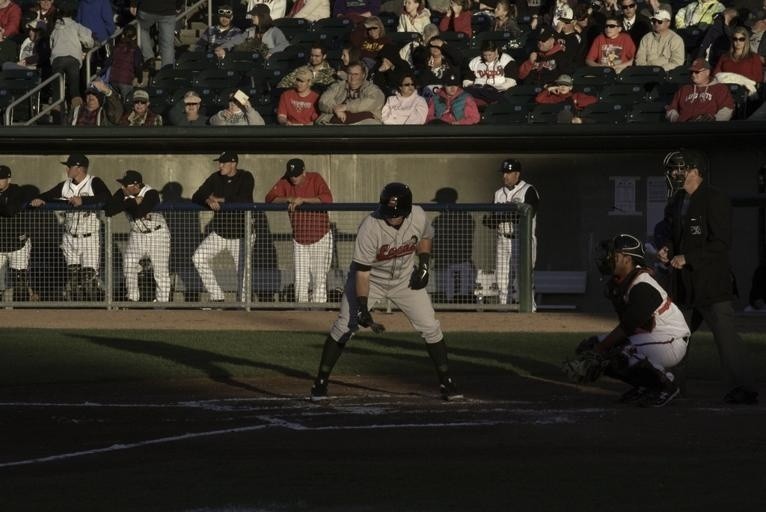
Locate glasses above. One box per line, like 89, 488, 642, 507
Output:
294, 0, 766, 107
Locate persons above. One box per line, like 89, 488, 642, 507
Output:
105, 170, 170, 310
576, 233, 691, 408
265, 158, 333, 312
31, 151, 113, 308
191, 151, 257, 312
643, 148, 759, 405
0, 165, 31, 310
0, 0, 766, 126
482, 158, 541, 313
309, 182, 464, 402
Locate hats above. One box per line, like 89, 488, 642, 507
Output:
246, 4, 269, 15
61, 153, 89, 168
217, 3, 233, 18
213, 151, 239, 163
284, 158, 305, 178
82, 89, 104, 107
1, 165, 11, 180
430, 187, 458, 203
116, 170, 142, 186
132, 90, 148, 103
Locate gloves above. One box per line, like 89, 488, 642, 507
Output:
356, 295, 374, 329
411, 252, 430, 290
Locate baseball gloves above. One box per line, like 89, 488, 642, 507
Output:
575, 336, 602, 385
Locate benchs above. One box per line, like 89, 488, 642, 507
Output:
474, 270, 589, 306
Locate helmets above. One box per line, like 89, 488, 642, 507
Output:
498, 158, 522, 173
377, 183, 413, 219
663, 147, 707, 193
594, 232, 645, 278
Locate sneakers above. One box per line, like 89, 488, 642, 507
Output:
309, 376, 331, 403
437, 376, 464, 404
618, 379, 681, 409
